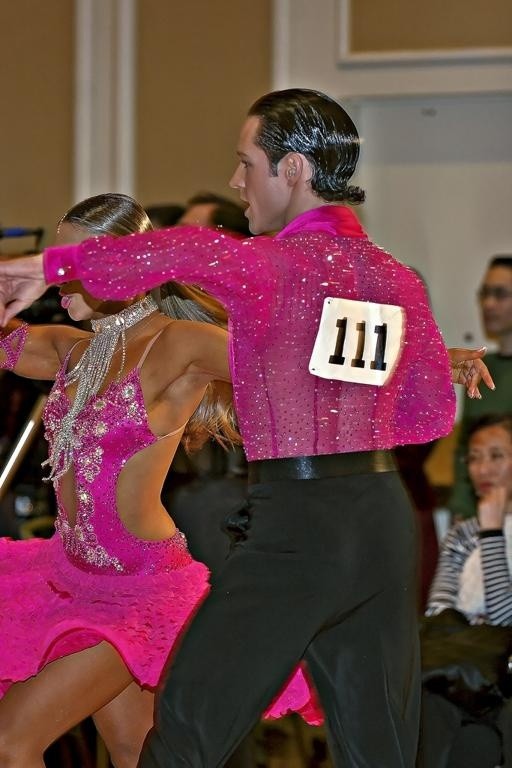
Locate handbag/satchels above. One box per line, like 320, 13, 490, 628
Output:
418, 610, 511, 713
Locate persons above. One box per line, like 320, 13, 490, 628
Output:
415, 414, 511, 767
0, 192, 495, 767
1, 89, 456, 768
2, 191, 510, 615
